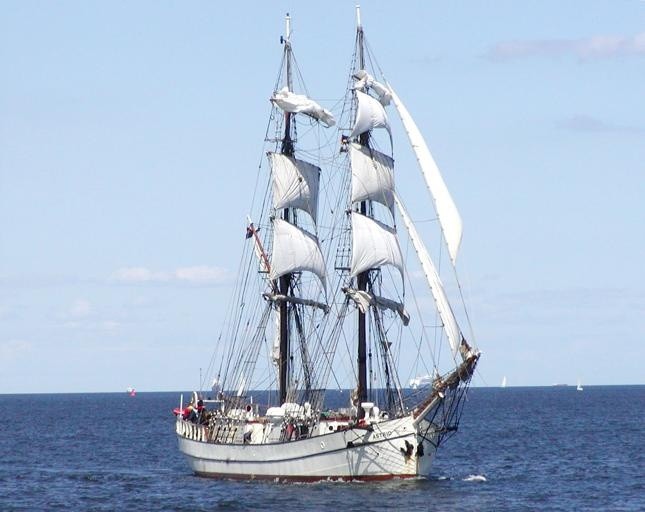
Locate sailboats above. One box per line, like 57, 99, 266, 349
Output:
168, 3, 486, 484
